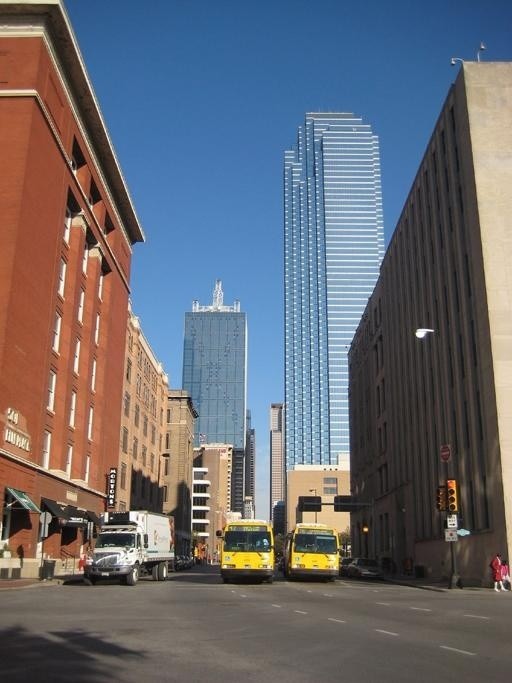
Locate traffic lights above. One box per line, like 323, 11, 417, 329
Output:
362, 519, 369, 534
446, 479, 458, 512
436, 487, 446, 512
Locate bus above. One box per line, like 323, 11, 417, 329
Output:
214, 518, 341, 584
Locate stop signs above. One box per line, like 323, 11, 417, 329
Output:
439, 445, 452, 463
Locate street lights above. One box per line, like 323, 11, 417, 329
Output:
308, 489, 318, 524
155, 453, 171, 511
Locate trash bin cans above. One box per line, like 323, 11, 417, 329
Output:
415, 565, 424, 578
43, 560, 56, 580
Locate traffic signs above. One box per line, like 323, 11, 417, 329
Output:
446, 515, 458, 529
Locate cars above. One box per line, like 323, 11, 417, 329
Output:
173, 554, 195, 571
339, 557, 384, 581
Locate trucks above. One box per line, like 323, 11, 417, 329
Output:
83, 509, 175, 586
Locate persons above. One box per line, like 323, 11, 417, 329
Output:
500, 559, 511, 587
17, 541, 25, 567
489, 551, 508, 592
